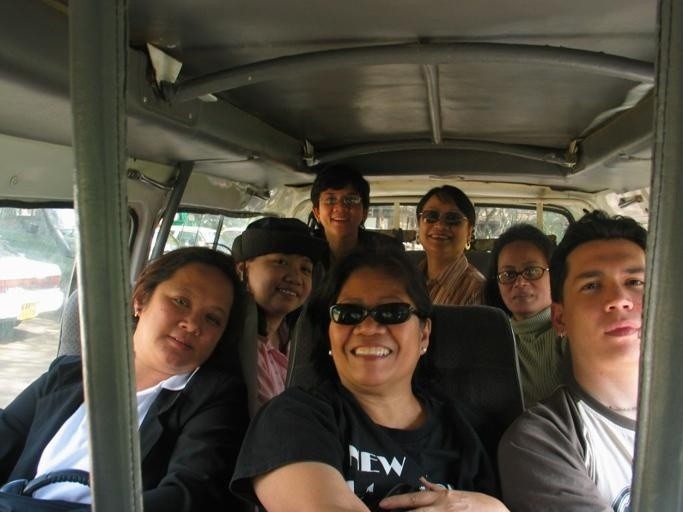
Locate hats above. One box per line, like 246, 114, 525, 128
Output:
232, 216, 320, 265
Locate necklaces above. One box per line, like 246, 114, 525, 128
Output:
604, 400, 641, 413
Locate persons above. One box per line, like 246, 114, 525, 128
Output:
228, 243, 510, 512
496, 210, 648, 512
409, 184, 487, 309
306, 161, 407, 302
0, 246, 246, 511
492, 224, 575, 413
216, 216, 316, 414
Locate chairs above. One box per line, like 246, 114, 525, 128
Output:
285, 299, 526, 447
56, 284, 260, 427
405, 251, 497, 280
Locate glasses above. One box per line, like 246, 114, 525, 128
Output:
497, 266, 550, 285
418, 210, 468, 225
329, 302, 426, 326
319, 196, 362, 207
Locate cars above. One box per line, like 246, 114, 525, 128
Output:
148, 226, 177, 259
0, 235, 65, 337
170, 224, 231, 255
219, 227, 246, 248
